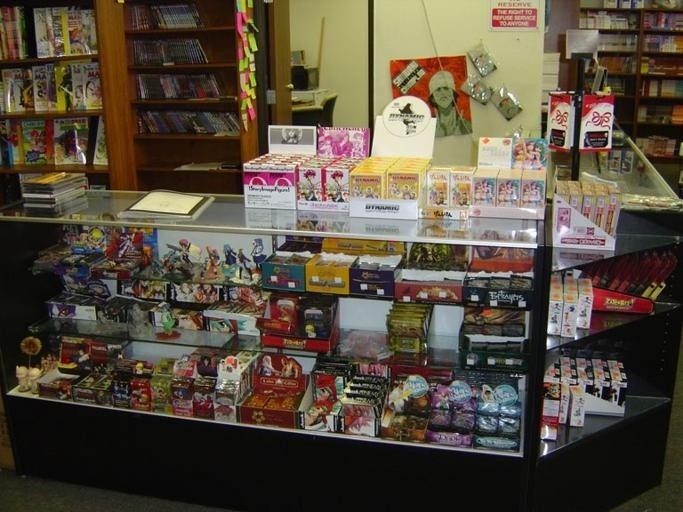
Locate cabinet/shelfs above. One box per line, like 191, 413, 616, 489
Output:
0, 1, 682, 512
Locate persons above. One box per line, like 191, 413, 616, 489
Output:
426, 69, 472, 139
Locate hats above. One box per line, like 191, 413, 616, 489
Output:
428, 71, 454, 94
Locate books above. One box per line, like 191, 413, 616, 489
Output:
130, 3, 205, 32
137, 110, 245, 134
19, 172, 88, 217
0, 5, 28, 60
133, 39, 209, 65
0, 116, 109, 166
574, 249, 679, 304
575, 0, 682, 161
116, 189, 216, 220
0, 60, 103, 113
33, 5, 98, 58
135, 72, 226, 99
